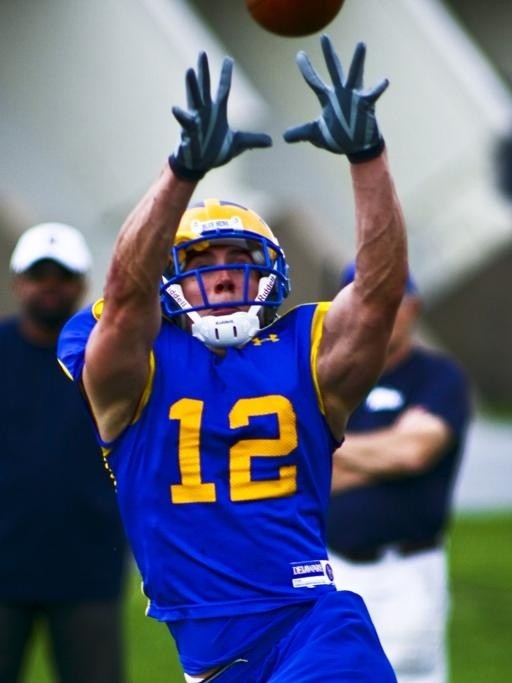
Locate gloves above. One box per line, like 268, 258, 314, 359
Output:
282, 31, 391, 165
165, 47, 275, 185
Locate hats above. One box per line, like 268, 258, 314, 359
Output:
8, 219, 94, 276
336, 258, 419, 297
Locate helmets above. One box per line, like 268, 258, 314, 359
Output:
163, 196, 295, 351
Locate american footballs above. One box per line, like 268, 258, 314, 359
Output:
245, 0, 344, 37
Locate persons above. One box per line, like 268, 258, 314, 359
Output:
53, 28, 409, 683
323, 256, 473, 682
0, 221, 138, 683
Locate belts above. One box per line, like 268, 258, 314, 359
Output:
331, 532, 446, 564
